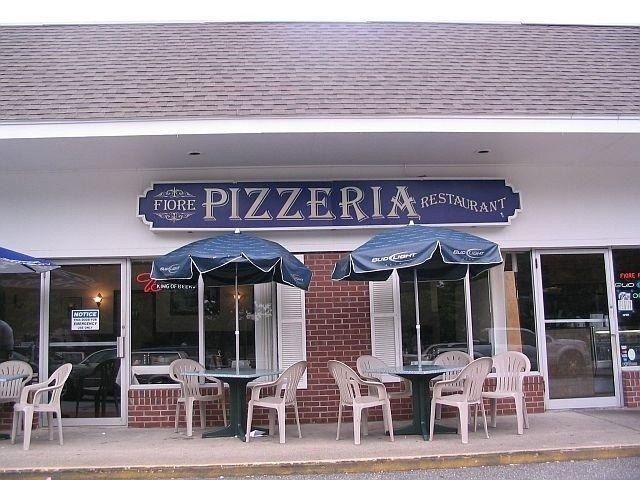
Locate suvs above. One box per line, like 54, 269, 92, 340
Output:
47, 346, 120, 402
545, 333, 592, 379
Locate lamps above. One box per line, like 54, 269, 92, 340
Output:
233, 291, 241, 304
93, 293, 104, 308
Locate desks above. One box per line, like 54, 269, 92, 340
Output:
365, 365, 466, 441
180, 369, 283, 442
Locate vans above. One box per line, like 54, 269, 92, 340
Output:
130, 349, 190, 384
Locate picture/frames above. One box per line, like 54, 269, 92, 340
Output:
169, 287, 220, 317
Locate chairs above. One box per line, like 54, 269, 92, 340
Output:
246, 360, 308, 443
429, 351, 473, 424
356, 355, 412, 435
326, 360, 394, 445
76, 359, 121, 418
473, 351, 531, 434
169, 358, 227, 437
430, 357, 493, 444
0, 360, 33, 435
11, 363, 73, 450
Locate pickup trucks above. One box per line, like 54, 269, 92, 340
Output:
422, 328, 537, 372
